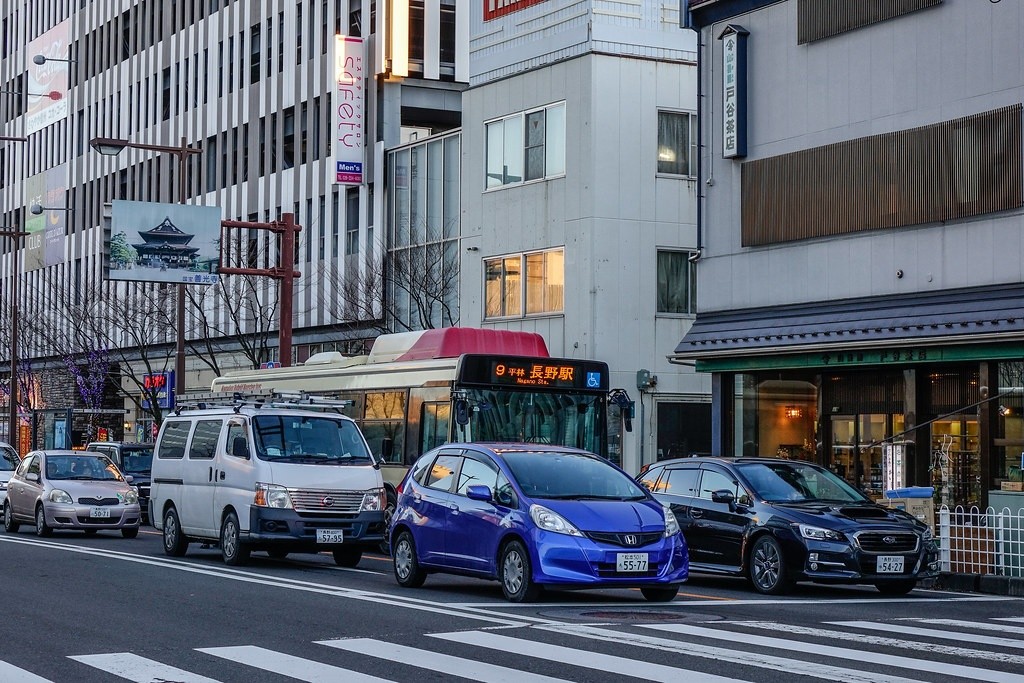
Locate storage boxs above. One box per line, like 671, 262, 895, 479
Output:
879, 497, 936, 546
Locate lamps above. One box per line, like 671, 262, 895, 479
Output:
784, 385, 804, 418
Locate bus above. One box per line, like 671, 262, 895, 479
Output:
210, 328, 636, 561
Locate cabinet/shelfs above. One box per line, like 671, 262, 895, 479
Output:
832, 441, 988, 512
983, 489, 1024, 578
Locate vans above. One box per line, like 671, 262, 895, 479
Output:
146, 389, 389, 569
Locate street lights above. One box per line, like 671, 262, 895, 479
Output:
88, 132, 203, 396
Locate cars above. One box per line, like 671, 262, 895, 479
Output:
3, 448, 142, 539
382, 440, 691, 604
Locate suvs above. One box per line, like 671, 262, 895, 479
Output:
626, 450, 940, 600
0, 442, 23, 521
84, 441, 182, 528
